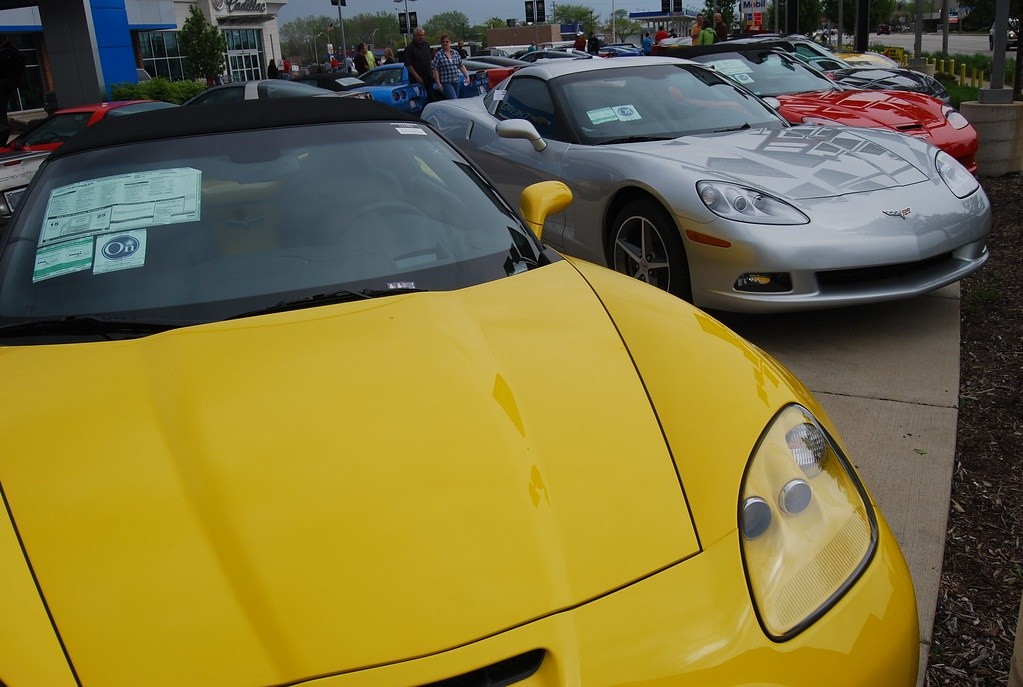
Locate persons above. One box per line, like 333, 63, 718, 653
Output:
403, 27, 433, 90
346, 55, 352, 72
588, 31, 599, 56
354, 42, 394, 74
331, 57, 338, 72
282, 55, 290, 80
267, 59, 278, 78
641, 13, 728, 55
573, 30, 585, 51
456, 40, 468, 59
528, 41, 535, 51
541, 43, 546, 57
433, 33, 469, 100
431, 47, 438, 60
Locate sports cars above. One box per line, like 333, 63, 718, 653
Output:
182, 79, 375, 102
358, 44, 646, 97
293, 73, 429, 116
0, 97, 922, 687
421, 58, 991, 317
649, 46, 979, 174
719, 34, 951, 105
1, 100, 180, 225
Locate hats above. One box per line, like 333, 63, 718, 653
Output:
577, 31, 585, 36
669, 28, 674, 32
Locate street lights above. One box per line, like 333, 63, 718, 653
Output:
314, 34, 321, 64
394, 0, 416, 43
331, 0, 349, 74
321, 32, 332, 61
373, 29, 379, 45
588, 9, 593, 32
388, 8, 398, 45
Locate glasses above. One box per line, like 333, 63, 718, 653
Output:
441, 41, 449, 43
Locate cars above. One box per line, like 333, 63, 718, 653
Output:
290, 62, 345, 79
137, 69, 151, 84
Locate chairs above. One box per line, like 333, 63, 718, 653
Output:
282, 166, 389, 244
